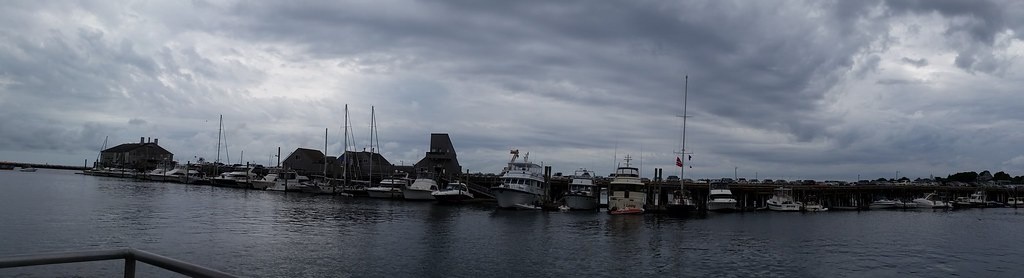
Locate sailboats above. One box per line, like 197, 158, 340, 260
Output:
666, 75, 697, 220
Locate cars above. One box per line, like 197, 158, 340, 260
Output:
640, 178, 650, 181
697, 178, 759, 183
667, 176, 680, 182
762, 179, 773, 183
652, 177, 663, 181
774, 180, 788, 183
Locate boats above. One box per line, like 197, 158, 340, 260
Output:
401, 178, 440, 202
766, 187, 800, 212
869, 191, 1024, 210
431, 180, 474, 203
797, 201, 828, 212
83, 104, 374, 198
608, 155, 647, 213
366, 179, 408, 199
20, 168, 38, 172
490, 152, 550, 208
705, 181, 737, 212
565, 168, 598, 212
